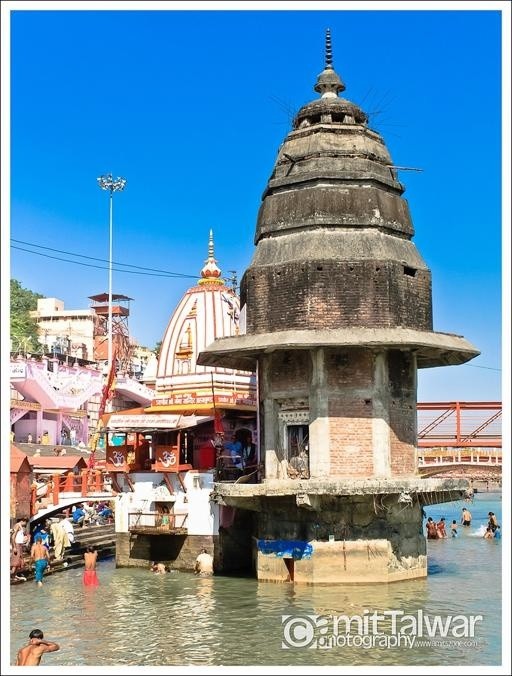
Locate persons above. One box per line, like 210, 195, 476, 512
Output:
223, 435, 244, 470
16, 629, 59, 666
195, 549, 214, 576
11, 501, 113, 587
448, 520, 457, 538
243, 437, 257, 466
426, 517, 437, 539
483, 512, 500, 540
437, 518, 447, 538
462, 508, 472, 526
28, 429, 85, 456
